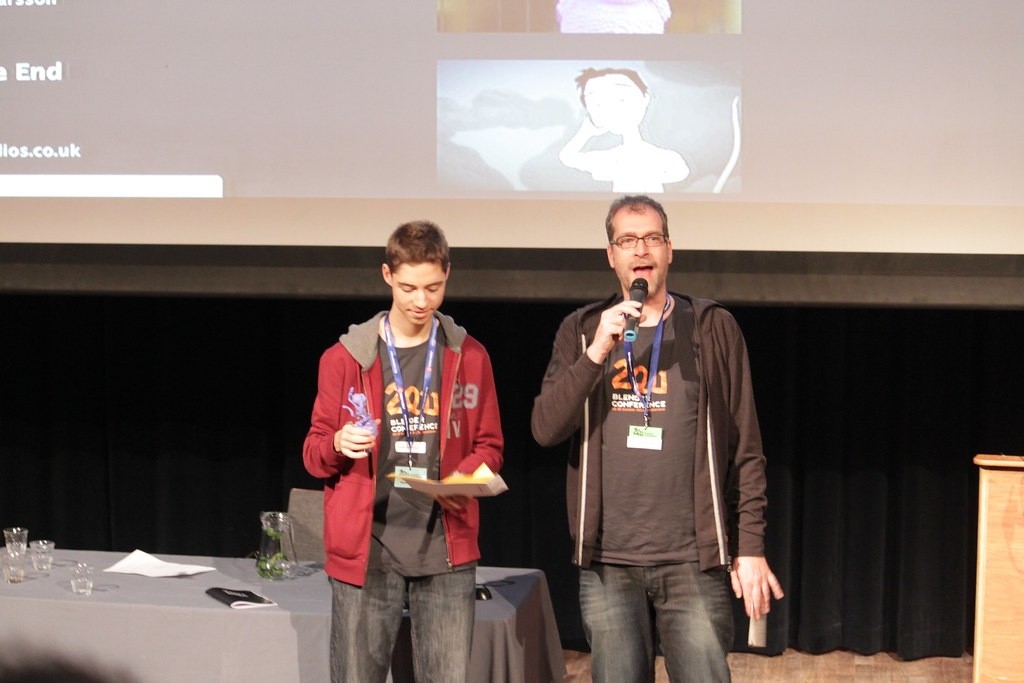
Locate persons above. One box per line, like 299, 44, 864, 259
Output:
533, 197, 784, 683
303, 222, 504, 683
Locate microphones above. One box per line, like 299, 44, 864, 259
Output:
623, 278, 648, 342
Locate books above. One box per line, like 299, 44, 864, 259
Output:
206, 588, 278, 609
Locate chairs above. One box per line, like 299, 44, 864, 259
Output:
286, 488, 327, 563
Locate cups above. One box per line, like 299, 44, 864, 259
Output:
3, 527, 28, 556
29, 540, 55, 573
3, 554, 25, 584
70, 561, 94, 596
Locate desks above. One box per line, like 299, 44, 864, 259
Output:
0, 544, 571, 683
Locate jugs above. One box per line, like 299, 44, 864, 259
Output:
256, 510, 299, 582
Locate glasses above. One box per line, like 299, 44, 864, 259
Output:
610, 232, 667, 249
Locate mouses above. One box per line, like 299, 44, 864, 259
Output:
476, 587, 492, 601
